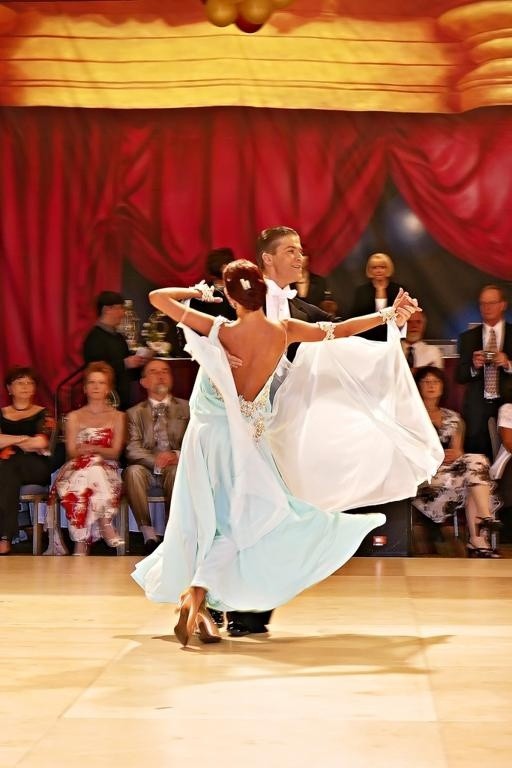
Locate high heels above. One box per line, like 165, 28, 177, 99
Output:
474, 517, 503, 536
465, 541, 500, 558
174, 588, 222, 645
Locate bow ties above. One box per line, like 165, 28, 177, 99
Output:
266, 281, 298, 300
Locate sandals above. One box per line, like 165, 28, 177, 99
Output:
70, 524, 125, 555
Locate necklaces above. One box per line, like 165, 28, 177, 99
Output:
12, 403, 32, 411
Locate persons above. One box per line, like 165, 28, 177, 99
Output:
83, 292, 144, 410
131, 256, 445, 645
43, 361, 126, 557
289, 244, 327, 306
352, 253, 408, 342
409, 364, 501, 558
179, 226, 420, 636
183, 245, 242, 322
453, 285, 512, 457
122, 357, 191, 557
400, 310, 443, 368
0, 368, 61, 554
494, 403, 512, 518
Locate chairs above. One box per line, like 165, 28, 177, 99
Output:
18, 364, 169, 555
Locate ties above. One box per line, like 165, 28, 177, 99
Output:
483, 330, 500, 395
406, 346, 414, 368
156, 403, 169, 450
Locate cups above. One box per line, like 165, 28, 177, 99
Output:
484, 353, 495, 365
148, 343, 169, 355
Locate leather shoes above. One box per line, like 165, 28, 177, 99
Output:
227, 619, 249, 636
144, 536, 162, 555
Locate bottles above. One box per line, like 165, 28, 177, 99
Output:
118, 299, 138, 348
